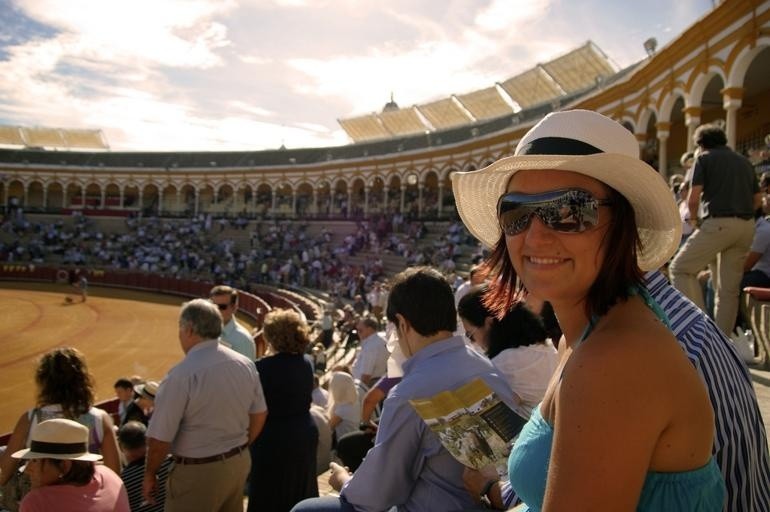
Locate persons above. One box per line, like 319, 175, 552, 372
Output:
449, 110, 729, 512
665, 122, 770, 336
0, 266, 560, 512
639, 270, 770, 512
1, 195, 482, 303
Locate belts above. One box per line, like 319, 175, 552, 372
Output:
701, 210, 755, 221
174, 438, 251, 467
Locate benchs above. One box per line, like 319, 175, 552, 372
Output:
0, 211, 241, 287
739, 286, 770, 376
240, 217, 488, 384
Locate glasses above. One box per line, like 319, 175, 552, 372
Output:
463, 322, 486, 346
217, 301, 234, 310
495, 185, 618, 238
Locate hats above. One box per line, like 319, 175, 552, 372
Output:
133, 381, 160, 401
10, 417, 104, 462
451, 108, 683, 273
